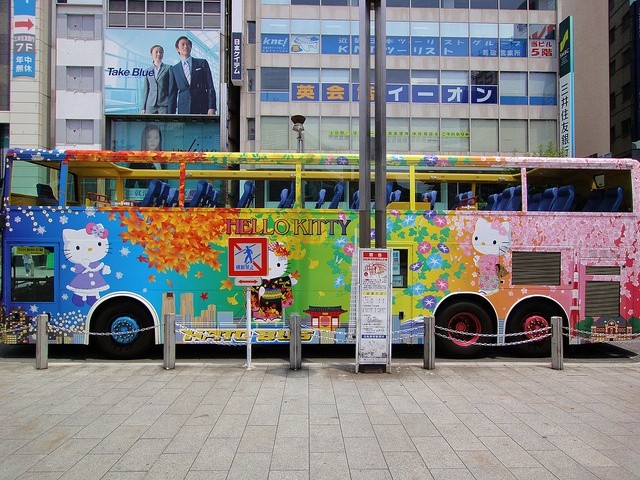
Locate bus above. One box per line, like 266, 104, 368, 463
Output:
0, 153, 639, 359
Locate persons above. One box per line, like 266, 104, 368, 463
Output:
141, 45, 172, 114
125, 125, 169, 188
168, 36, 217, 115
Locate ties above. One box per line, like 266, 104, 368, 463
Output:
184, 61, 191, 85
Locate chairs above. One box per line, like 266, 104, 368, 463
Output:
484, 194, 498, 211
461, 192, 472, 205
598, 186, 623, 212
583, 190, 600, 211
392, 191, 402, 202
282, 181, 295, 208
420, 193, 429, 201
450, 194, 462, 209
529, 193, 543, 212
139, 179, 161, 207
349, 191, 359, 210
165, 188, 178, 207
373, 191, 392, 209
314, 189, 326, 208
328, 182, 344, 209
551, 185, 575, 212
191, 181, 207, 207
35, 183, 59, 206
237, 181, 253, 207
493, 193, 502, 210
428, 191, 437, 209
506, 186, 522, 210
495, 188, 515, 211
154, 183, 170, 207
199, 184, 213, 207
538, 189, 558, 211
277, 189, 289, 206
209, 190, 221, 207
